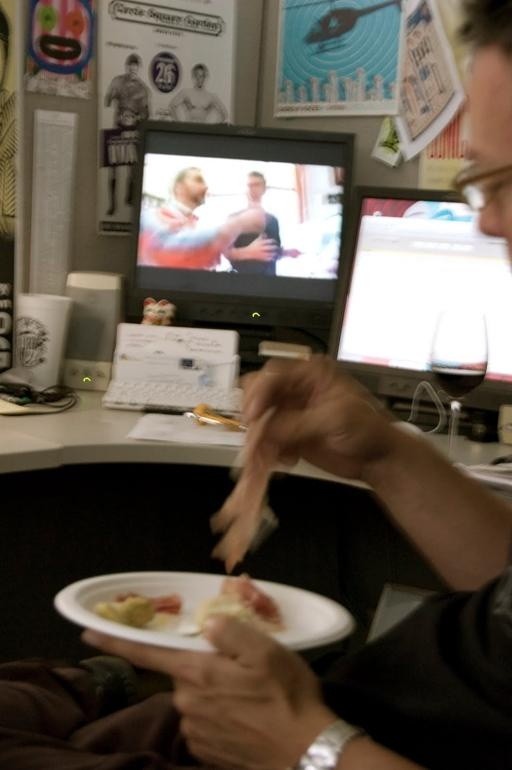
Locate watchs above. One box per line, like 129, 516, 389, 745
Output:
295, 718, 363, 770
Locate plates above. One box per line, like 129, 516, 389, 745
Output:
52, 569, 359, 661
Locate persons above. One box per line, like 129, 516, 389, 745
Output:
103, 52, 150, 215
138, 166, 277, 271
82, 2, 512, 770
171, 61, 230, 125
218, 172, 300, 275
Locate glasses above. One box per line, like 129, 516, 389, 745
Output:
452, 164, 512, 209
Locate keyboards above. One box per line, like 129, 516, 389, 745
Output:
101, 378, 245, 416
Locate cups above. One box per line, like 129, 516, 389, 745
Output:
422, 304, 493, 463
9, 292, 77, 396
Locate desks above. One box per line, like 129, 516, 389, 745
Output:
0, 359, 510, 516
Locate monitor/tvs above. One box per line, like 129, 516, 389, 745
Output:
324, 183, 511, 428
125, 117, 356, 368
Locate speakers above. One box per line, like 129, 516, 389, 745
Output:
60, 269, 123, 391
